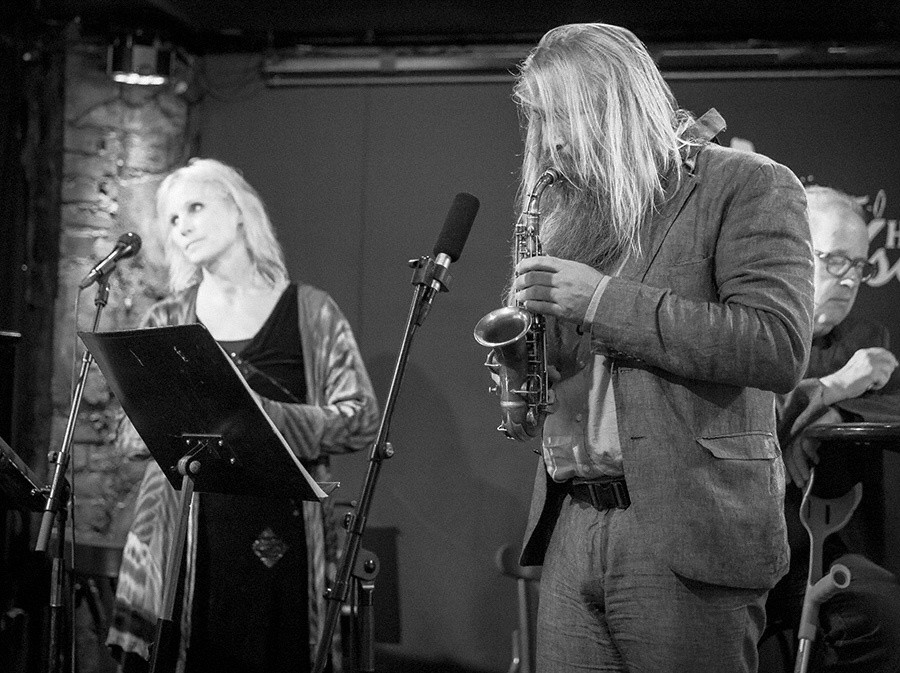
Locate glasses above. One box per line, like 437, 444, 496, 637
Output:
813, 249, 875, 283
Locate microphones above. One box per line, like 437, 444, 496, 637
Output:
415, 192, 479, 326
78, 231, 143, 290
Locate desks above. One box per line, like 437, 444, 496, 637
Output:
807, 422, 900, 439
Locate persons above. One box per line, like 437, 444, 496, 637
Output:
773, 185, 900, 673
485, 22, 815, 673
105, 156, 382, 673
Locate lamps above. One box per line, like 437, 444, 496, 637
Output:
108, 35, 175, 88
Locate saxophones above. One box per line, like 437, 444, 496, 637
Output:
474, 167, 562, 443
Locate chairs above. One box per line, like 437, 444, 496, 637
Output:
495, 543, 543, 673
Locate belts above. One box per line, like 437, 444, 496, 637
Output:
566, 479, 632, 512
